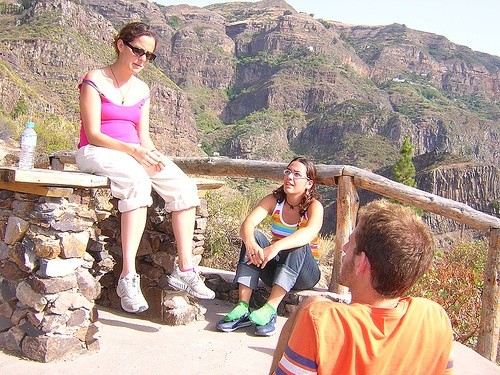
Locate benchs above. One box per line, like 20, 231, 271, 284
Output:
1, 167, 224, 359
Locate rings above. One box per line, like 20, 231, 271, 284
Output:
251, 254, 256, 257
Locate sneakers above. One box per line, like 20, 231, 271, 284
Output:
116, 273, 149, 313
216, 308, 252, 331
166, 262, 215, 299
255, 313, 277, 335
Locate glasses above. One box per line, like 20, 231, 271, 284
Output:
284, 169, 310, 180
125, 42, 156, 60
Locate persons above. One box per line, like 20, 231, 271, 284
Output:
78, 22, 215, 313
223, 157, 324, 326
270, 197, 454, 375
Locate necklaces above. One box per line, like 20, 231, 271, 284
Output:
109, 65, 133, 105
284, 199, 302, 210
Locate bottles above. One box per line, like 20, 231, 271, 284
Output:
19, 123, 38, 170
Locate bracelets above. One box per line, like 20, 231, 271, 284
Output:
151, 149, 155, 152
